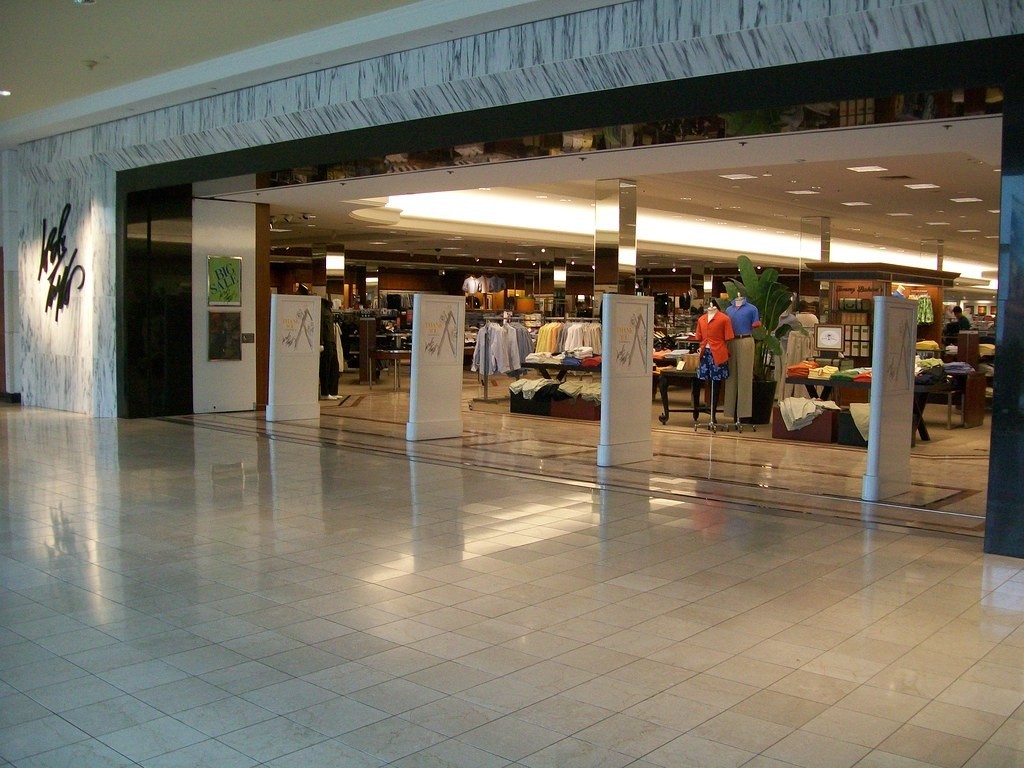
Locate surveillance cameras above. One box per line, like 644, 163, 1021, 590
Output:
435, 248, 441, 252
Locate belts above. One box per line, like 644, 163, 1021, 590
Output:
734, 335, 751, 338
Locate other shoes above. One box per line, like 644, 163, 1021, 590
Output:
321, 393, 343, 400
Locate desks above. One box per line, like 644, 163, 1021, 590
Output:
521, 359, 721, 425
785, 364, 988, 442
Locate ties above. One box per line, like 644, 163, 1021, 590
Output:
484, 278, 488, 292
495, 278, 498, 289
474, 279, 476, 292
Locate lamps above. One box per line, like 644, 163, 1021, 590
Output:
531, 260, 536, 265
410, 251, 414, 257
284, 214, 293, 222
303, 214, 311, 219
270, 216, 278, 224
475, 256, 480, 262
498, 256, 502, 264
546, 259, 550, 265
436, 252, 441, 259
514, 255, 519, 261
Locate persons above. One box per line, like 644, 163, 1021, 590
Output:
319, 299, 343, 400
695, 306, 735, 380
462, 274, 507, 295
774, 310, 803, 381
950, 306, 971, 346
295, 284, 309, 295
892, 285, 906, 298
723, 297, 761, 418
678, 292, 691, 310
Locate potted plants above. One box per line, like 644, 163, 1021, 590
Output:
716, 255, 808, 424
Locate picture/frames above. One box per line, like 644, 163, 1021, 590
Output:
814, 323, 844, 351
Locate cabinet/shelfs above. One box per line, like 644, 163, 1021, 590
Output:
944, 334, 996, 410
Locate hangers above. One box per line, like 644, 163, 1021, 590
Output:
486, 317, 523, 326
544, 319, 600, 324
